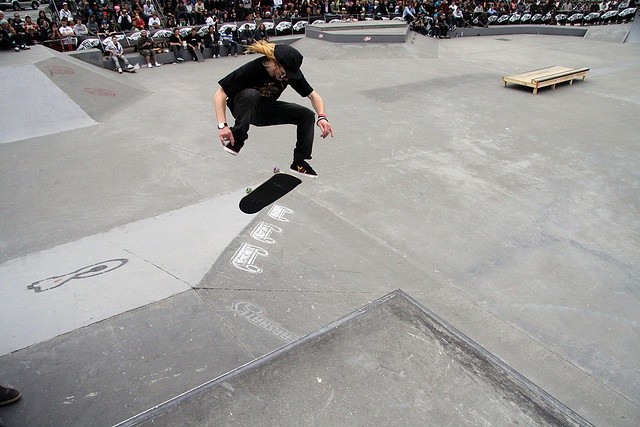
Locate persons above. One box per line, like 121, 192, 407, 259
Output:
158, 0, 271, 26
186, 28, 204, 61
240, 24, 254, 54
271, 0, 450, 37
450, 0, 640, 30
105, 36, 134, 73
213, 41, 334, 178
1, 0, 160, 50
204, 25, 219, 58
220, 27, 238, 57
168, 28, 184, 64
137, 31, 160, 68
254, 24, 269, 42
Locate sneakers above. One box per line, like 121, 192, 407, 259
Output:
127, 64, 134, 69
118, 67, 123, 73
177, 57, 184, 60
289, 160, 318, 179
154, 62, 161, 67
0, 385, 21, 406
224, 139, 245, 155
148, 63, 152, 68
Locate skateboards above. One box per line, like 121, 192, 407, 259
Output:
238, 168, 302, 216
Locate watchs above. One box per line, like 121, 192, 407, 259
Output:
217, 123, 228, 129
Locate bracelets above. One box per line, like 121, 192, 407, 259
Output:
316, 114, 330, 126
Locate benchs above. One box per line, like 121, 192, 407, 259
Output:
501, 64, 590, 95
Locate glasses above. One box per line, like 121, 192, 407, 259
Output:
278, 66, 287, 78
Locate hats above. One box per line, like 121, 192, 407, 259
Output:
274, 44, 303, 79
